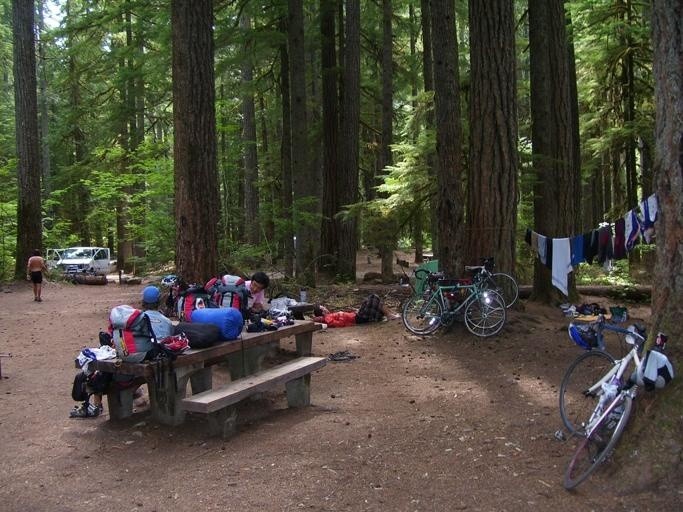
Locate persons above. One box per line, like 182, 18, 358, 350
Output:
27, 249, 48, 301
314, 294, 401, 327
245, 272, 269, 310
71, 286, 172, 417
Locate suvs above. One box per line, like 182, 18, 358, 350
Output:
45, 246, 111, 277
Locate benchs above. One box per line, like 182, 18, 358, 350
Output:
103, 375, 145, 422
179, 356, 328, 436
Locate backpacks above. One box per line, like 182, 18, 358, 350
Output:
110, 275, 248, 363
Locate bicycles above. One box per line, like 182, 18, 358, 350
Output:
555, 312, 649, 490
401, 255, 519, 338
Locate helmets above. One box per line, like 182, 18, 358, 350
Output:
570, 322, 603, 352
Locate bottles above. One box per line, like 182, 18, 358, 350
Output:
301, 288, 307, 301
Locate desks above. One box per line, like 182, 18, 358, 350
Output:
288, 301, 314, 320
89, 316, 324, 425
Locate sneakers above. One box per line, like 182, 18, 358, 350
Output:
70, 403, 103, 416
